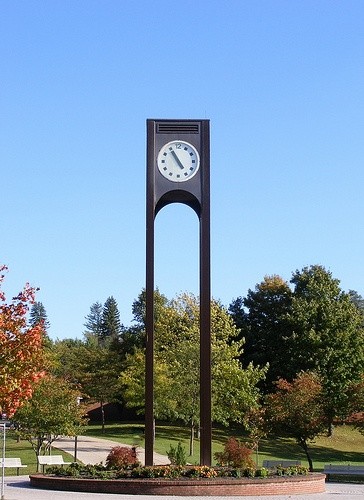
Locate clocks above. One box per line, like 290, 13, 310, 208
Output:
157, 141, 201, 182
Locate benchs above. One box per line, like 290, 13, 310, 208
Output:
37, 455, 77, 475
0, 457, 27, 477
322, 464, 364, 483
263, 460, 301, 468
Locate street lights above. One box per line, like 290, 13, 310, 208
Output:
74, 397, 83, 461
